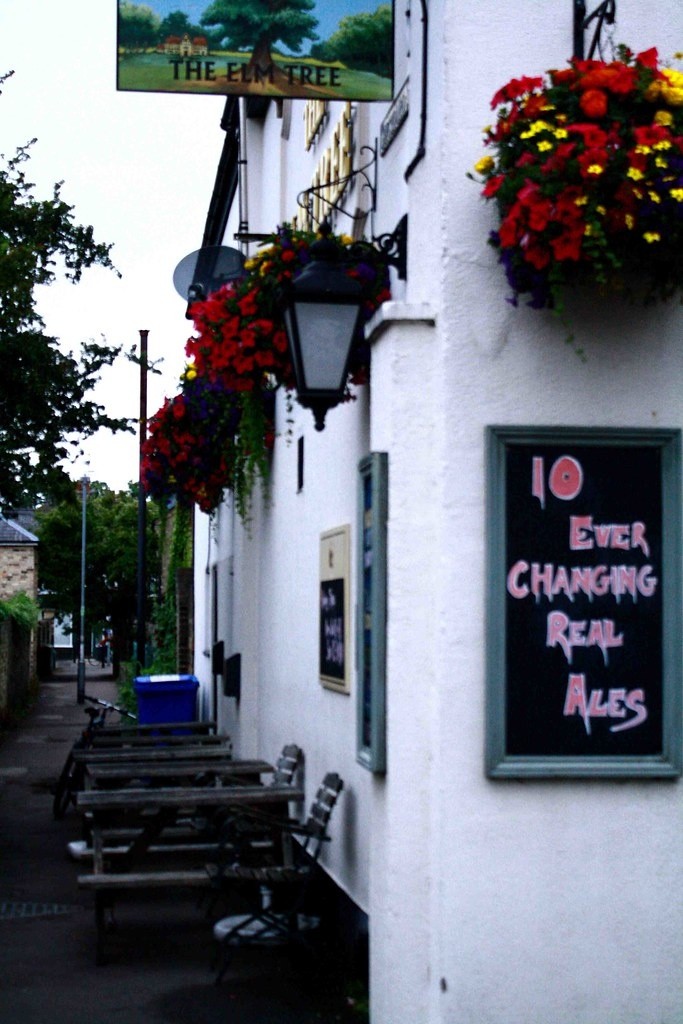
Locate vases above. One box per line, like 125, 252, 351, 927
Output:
278, 286, 362, 434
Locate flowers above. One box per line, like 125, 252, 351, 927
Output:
465, 38, 683, 367
138, 214, 391, 535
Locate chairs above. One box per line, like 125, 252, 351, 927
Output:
212, 771, 343, 950
215, 742, 303, 866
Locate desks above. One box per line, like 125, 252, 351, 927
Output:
69, 717, 305, 959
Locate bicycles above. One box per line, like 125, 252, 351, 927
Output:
89, 648, 110, 670
51, 696, 139, 819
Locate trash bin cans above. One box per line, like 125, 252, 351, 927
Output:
131, 674, 201, 748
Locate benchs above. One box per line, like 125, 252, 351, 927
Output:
82, 838, 278, 859
72, 863, 302, 895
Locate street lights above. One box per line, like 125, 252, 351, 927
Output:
78, 477, 108, 703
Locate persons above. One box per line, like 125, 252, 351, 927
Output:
95, 629, 113, 662
